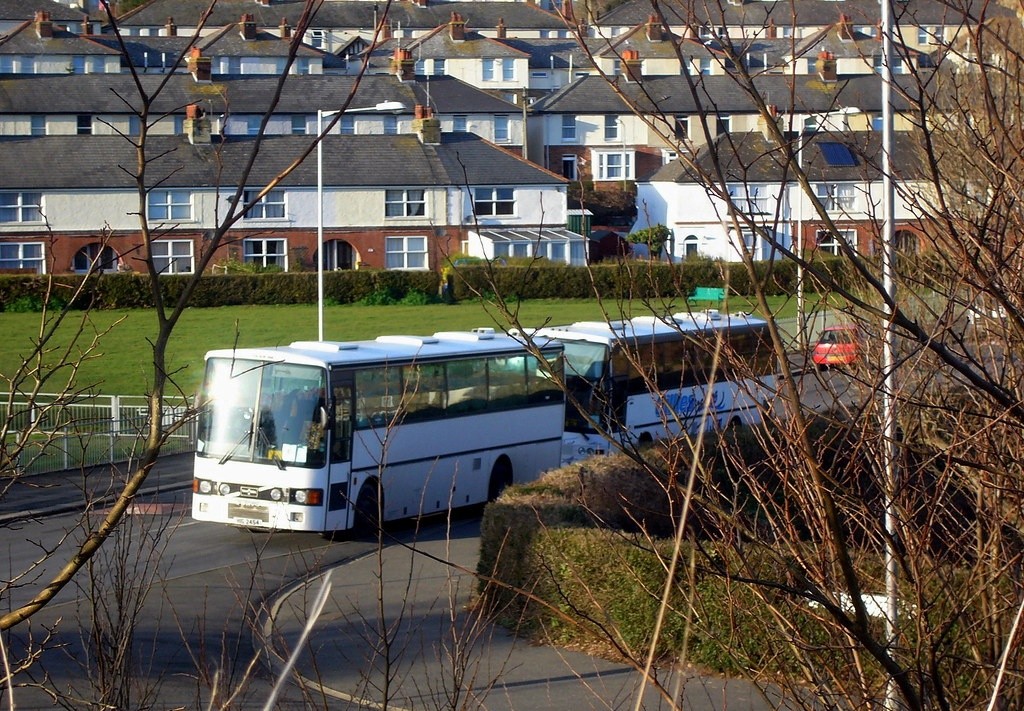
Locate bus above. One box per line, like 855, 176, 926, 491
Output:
191, 330, 567, 539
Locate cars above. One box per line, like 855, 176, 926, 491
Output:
510, 309, 779, 466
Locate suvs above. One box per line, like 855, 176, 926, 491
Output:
815, 326, 875, 370
968, 284, 1008, 339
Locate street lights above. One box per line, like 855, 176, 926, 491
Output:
317, 100, 407, 339
797, 107, 861, 351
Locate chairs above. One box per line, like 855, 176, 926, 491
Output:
256, 382, 396, 420
